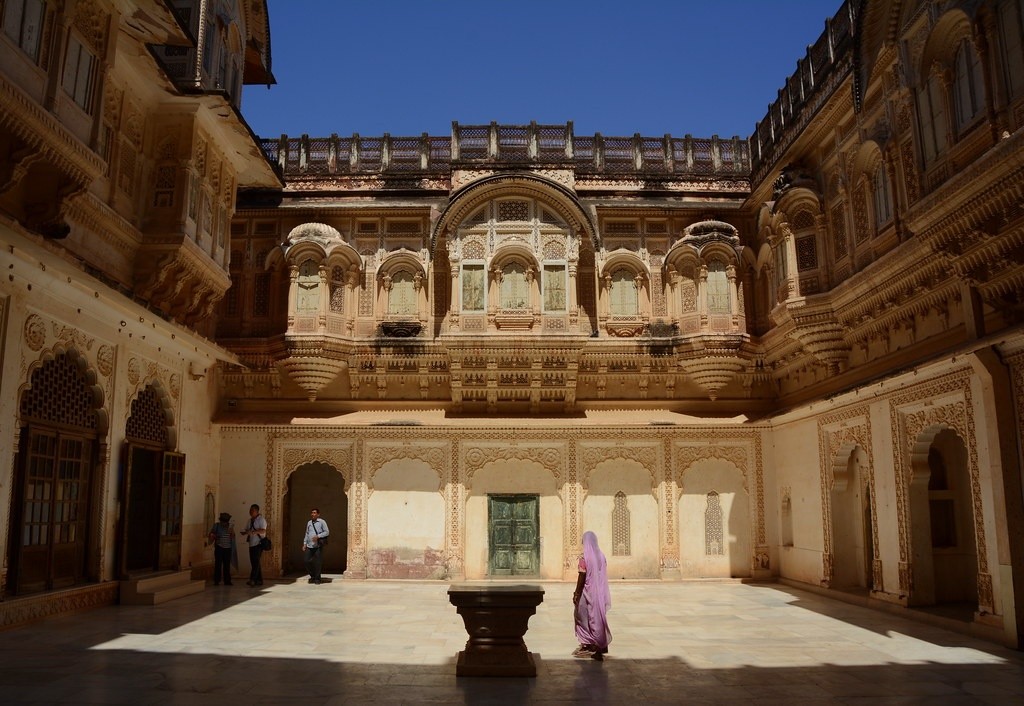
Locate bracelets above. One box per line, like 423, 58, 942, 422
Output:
573, 592, 582, 596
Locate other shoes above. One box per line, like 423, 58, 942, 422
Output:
214, 579, 221, 586
224, 582, 233, 585
591, 654, 604, 662
315, 578, 321, 584
255, 581, 263, 586
309, 576, 316, 583
246, 581, 254, 586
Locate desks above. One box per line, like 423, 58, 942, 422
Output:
447, 584, 545, 677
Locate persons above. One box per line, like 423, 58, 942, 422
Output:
302, 508, 330, 584
207, 512, 238, 587
571, 530, 613, 662
239, 503, 268, 587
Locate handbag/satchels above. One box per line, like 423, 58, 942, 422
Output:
261, 537, 272, 551
317, 536, 328, 546
